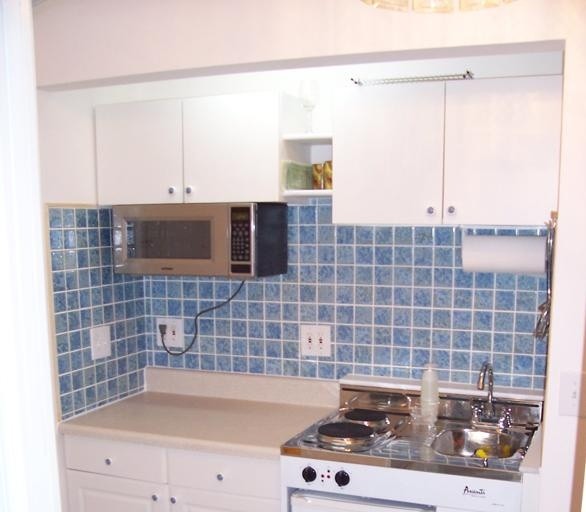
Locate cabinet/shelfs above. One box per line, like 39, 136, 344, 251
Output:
329, 75, 562, 228
61, 439, 282, 512
93, 101, 281, 204
280, 90, 333, 200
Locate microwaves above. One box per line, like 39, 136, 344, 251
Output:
110, 202, 289, 279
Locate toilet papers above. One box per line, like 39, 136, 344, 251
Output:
461, 236, 547, 275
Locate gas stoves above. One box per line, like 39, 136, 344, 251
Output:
285, 404, 410, 463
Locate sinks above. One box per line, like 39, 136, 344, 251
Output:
431, 429, 523, 459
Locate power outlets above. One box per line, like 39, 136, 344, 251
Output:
297, 325, 332, 357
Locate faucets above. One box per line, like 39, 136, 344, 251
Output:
477, 362, 494, 416
